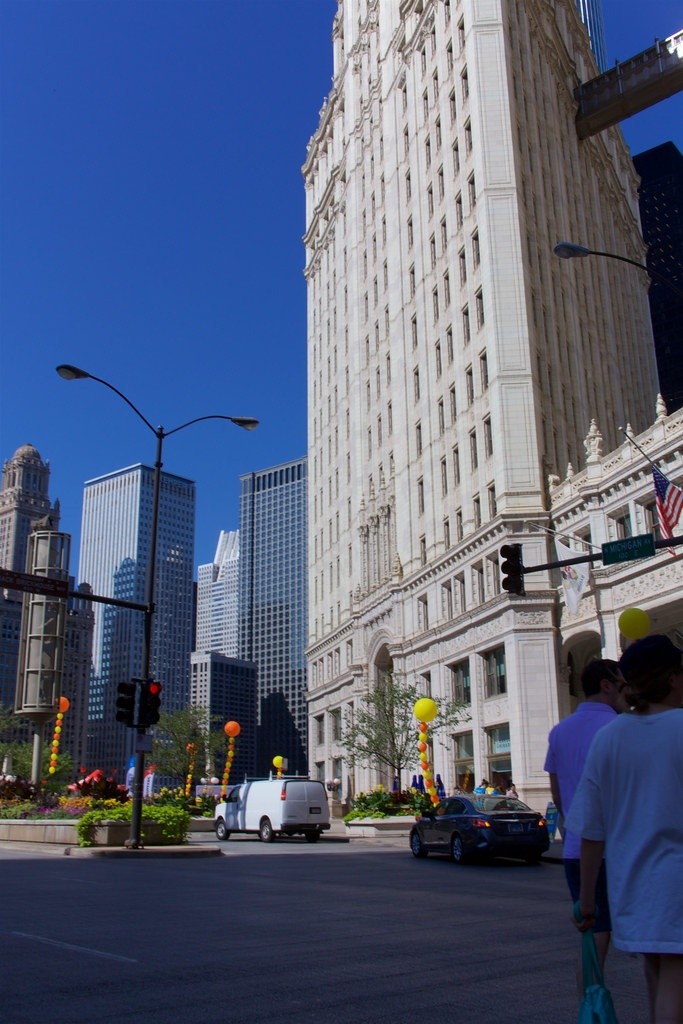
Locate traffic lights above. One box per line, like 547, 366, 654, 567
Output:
139, 681, 163, 728
115, 680, 135, 726
500, 543, 526, 594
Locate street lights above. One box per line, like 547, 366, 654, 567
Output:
55, 364, 262, 848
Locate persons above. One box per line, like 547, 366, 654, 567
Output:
474, 779, 518, 810
546, 635, 683, 1024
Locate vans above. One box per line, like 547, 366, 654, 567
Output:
214, 778, 330, 842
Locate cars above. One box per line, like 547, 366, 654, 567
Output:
409, 795, 550, 864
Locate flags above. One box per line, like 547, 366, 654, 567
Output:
552, 537, 590, 621
649, 464, 682, 564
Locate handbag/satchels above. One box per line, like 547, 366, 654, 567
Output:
572, 900, 619, 1024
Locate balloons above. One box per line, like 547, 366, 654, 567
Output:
413, 698, 447, 815
618, 608, 649, 640
272, 755, 284, 779
221, 721, 240, 805
47, 697, 68, 774
185, 743, 198, 799
